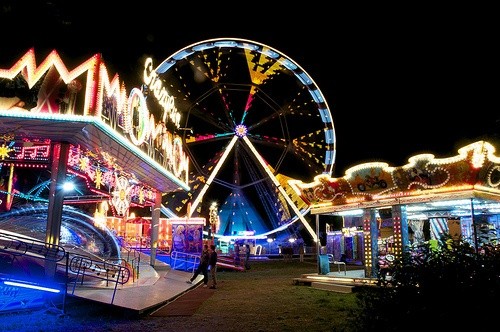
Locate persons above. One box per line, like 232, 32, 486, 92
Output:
208, 245, 218, 289
186, 245, 209, 288
234, 241, 251, 270
281, 243, 304, 264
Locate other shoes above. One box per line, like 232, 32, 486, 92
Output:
202, 284, 208, 288
209, 285, 216, 289
186, 280, 192, 284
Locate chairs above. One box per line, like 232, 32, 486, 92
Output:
327, 254, 346, 276
